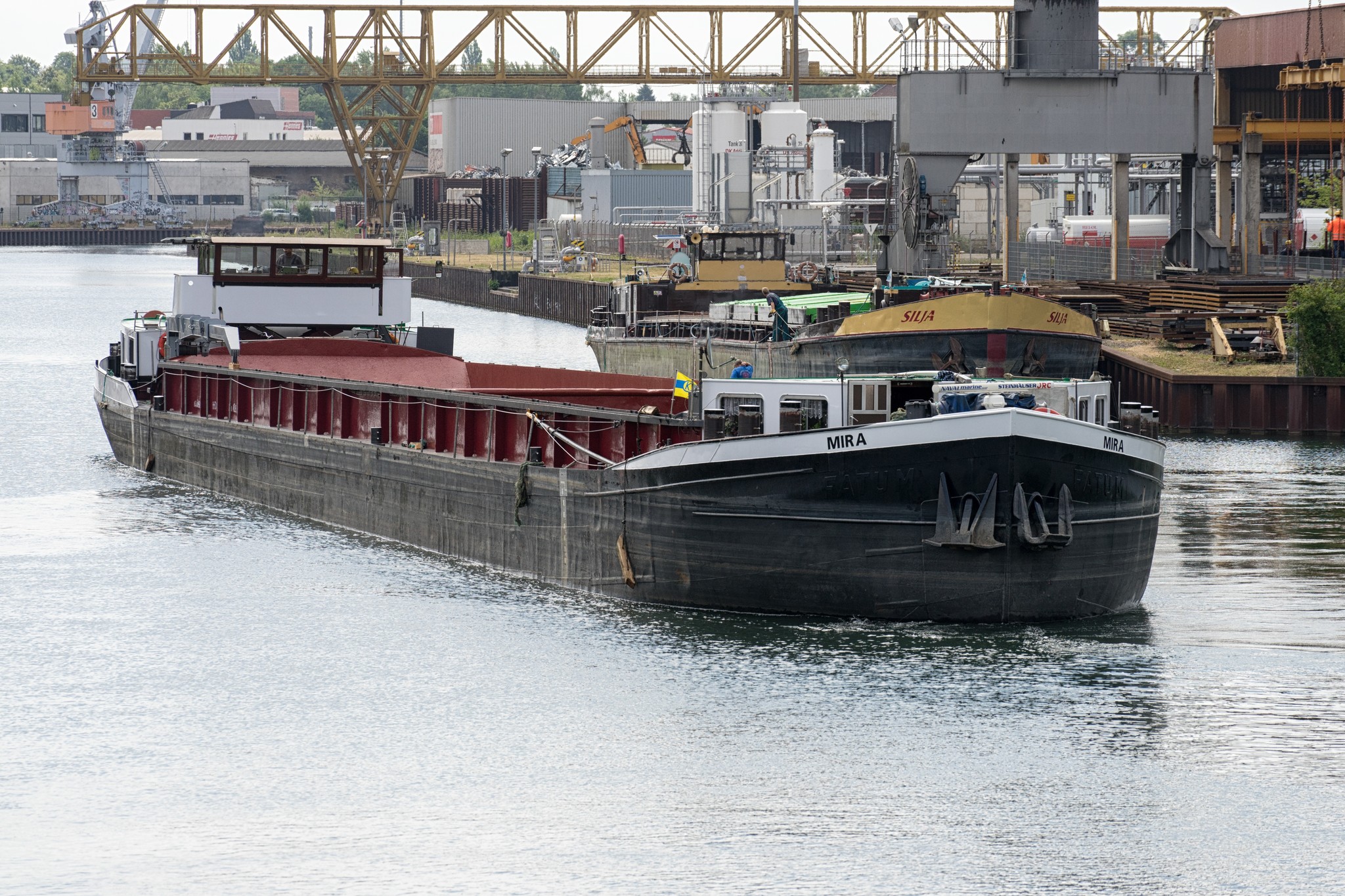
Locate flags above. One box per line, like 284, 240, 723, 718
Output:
674, 372, 699, 399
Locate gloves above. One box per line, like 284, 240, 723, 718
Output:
771, 309, 777, 316
768, 311, 773, 318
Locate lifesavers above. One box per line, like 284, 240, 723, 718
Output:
1033, 407, 1060, 416
158, 332, 166, 359
797, 261, 818, 282
667, 262, 688, 283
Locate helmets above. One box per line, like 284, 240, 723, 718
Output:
1334, 211, 1341, 215
350, 267, 359, 274
1285, 240, 1291, 245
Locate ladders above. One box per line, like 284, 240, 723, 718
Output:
391, 212, 411, 260
537, 218, 565, 276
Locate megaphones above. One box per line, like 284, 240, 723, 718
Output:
691, 233, 702, 244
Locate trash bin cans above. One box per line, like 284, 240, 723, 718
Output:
1293, 208, 1342, 249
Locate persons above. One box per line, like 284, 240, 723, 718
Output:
762, 287, 791, 343
1324, 211, 1345, 259
275, 248, 306, 274
730, 360, 753, 379
1280, 240, 1296, 277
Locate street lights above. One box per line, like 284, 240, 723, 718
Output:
1232, 155, 1241, 254
500, 148, 513, 230
531, 146, 543, 239
380, 155, 390, 238
362, 156, 373, 239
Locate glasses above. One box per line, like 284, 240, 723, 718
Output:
283, 249, 287, 250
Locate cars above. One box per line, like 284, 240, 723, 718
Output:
260, 208, 300, 223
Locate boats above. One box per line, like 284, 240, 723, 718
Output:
94, 237, 1172, 630
584, 226, 1111, 380
